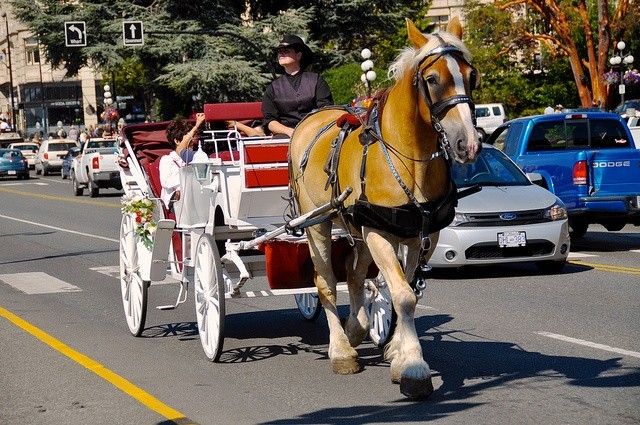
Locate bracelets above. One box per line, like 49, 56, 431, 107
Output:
186, 132, 194, 140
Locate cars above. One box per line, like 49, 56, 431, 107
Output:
608, 99, 640, 127
397, 141, 571, 276
0, 149, 29, 180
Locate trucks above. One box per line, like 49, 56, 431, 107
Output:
0, 131, 24, 148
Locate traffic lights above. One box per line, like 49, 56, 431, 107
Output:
69, 105, 84, 125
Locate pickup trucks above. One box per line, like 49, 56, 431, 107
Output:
485, 112, 640, 240
71, 135, 123, 198
7, 142, 40, 169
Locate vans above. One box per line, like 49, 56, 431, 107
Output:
473, 104, 507, 143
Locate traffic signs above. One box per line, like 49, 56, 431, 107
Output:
64, 21, 87, 46
123, 20, 144, 46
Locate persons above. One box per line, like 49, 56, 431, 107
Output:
224, 111, 267, 141
18, 123, 114, 144
261, 34, 334, 138
544, 104, 555, 114
554, 103, 563, 116
0, 118, 10, 129
145, 114, 151, 124
158, 109, 230, 262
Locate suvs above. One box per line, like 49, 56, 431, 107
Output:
34, 139, 79, 176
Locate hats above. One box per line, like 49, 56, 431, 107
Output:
272, 35, 314, 69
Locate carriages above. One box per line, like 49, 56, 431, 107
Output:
117, 15, 484, 403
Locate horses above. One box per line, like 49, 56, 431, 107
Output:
284, 16, 480, 402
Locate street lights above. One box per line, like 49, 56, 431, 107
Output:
103, 70, 119, 129
35, 27, 52, 133
0, 11, 16, 132
609, 37, 635, 103
